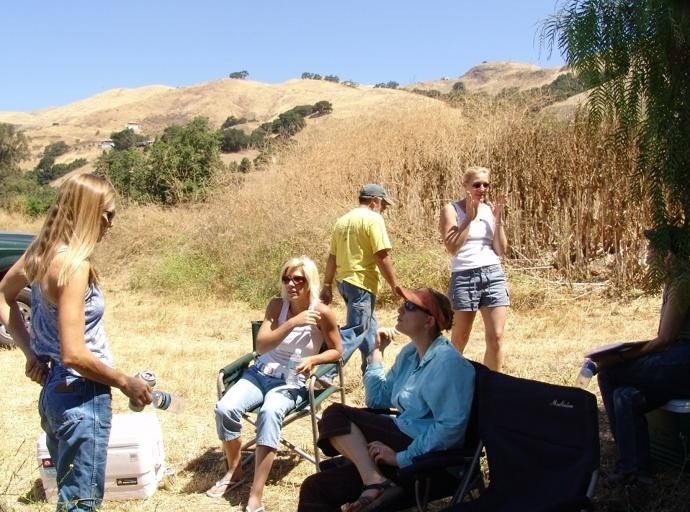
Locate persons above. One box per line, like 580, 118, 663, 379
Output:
206, 255, 344, 512
441, 167, 509, 371
0, 174, 153, 512
299, 284, 475, 511
319, 183, 401, 387
587, 224, 690, 483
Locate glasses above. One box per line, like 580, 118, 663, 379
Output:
467, 181, 489, 188
282, 274, 307, 285
403, 298, 432, 317
99, 206, 116, 220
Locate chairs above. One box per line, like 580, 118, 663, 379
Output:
217, 321, 344, 475
441, 371, 599, 512
319, 358, 489, 511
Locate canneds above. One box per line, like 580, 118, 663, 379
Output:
128, 369, 157, 412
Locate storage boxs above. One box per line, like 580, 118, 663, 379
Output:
37, 412, 166, 503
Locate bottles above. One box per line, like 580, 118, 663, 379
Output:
151, 391, 186, 414
575, 358, 597, 390
285, 348, 303, 389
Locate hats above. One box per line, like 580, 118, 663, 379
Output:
358, 184, 393, 205
395, 283, 448, 331
642, 222, 690, 256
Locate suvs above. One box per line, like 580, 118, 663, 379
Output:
0, 233, 40, 351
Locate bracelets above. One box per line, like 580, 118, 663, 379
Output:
495, 221, 505, 227
322, 283, 331, 287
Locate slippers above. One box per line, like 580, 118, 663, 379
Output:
244, 503, 265, 512
204, 469, 248, 500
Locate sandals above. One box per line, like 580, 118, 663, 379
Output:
337, 476, 407, 512
